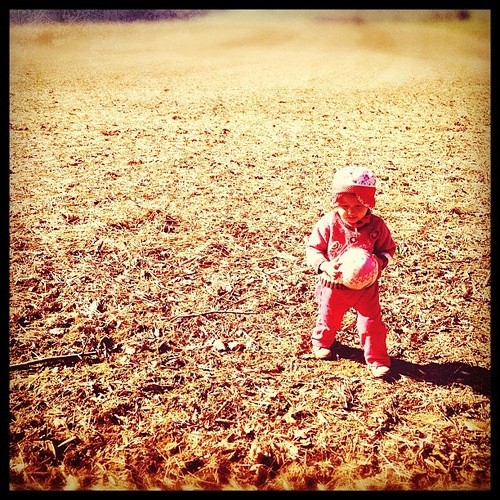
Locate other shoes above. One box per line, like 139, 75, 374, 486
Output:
367, 361, 392, 379
310, 341, 332, 360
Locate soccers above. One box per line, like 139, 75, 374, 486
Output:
336, 247, 377, 290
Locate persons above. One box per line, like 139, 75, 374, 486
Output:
307, 166, 397, 380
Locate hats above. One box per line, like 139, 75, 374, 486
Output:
329, 165, 377, 209
